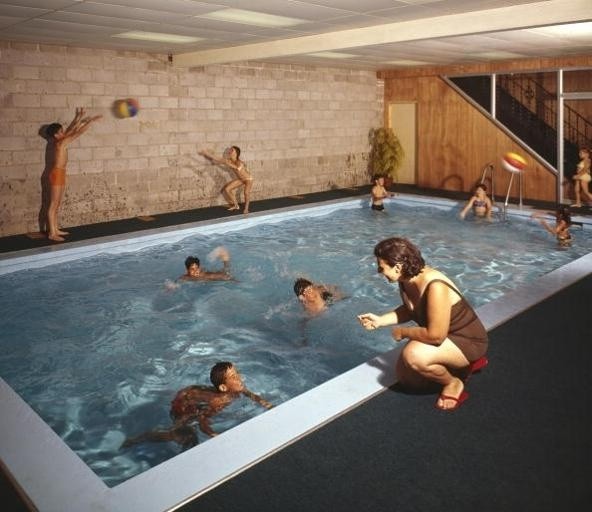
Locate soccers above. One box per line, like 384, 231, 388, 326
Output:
502, 151, 525, 173
119, 99, 136, 117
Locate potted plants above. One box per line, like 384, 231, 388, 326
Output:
367, 127, 404, 190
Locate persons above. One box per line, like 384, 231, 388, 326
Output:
294, 277, 349, 312
570, 148, 592, 207
536, 206, 573, 249
371, 173, 396, 211
357, 237, 490, 413
120, 361, 275, 453
199, 146, 253, 213
164, 255, 240, 297
458, 183, 493, 223
46, 106, 103, 241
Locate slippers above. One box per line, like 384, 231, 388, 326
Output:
434, 392, 470, 411
455, 356, 488, 384
